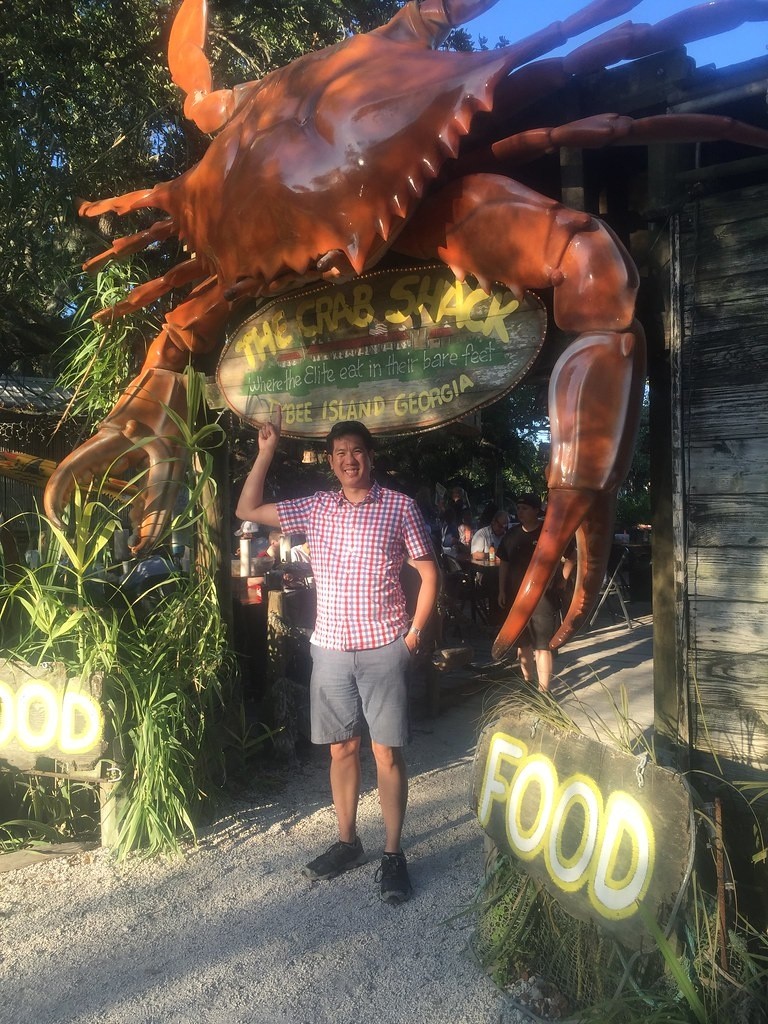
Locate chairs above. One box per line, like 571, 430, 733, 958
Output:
442, 542, 635, 647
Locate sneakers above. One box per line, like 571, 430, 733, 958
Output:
374, 848, 413, 905
304, 836, 368, 880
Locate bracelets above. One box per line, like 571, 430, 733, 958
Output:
409, 627, 423, 637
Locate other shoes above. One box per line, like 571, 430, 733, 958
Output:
532, 690, 553, 709
516, 680, 535, 695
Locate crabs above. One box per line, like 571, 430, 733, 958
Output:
44, 0, 768, 663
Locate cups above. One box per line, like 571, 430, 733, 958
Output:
303, 577, 314, 589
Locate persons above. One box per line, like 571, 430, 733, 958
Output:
24, 530, 183, 621
400, 488, 511, 621
233, 520, 316, 602
236, 407, 444, 904
495, 493, 576, 703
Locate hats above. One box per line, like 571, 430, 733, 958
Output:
516, 493, 537, 508
234, 520, 259, 536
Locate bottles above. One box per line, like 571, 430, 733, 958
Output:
488, 543, 496, 561
622, 530, 628, 544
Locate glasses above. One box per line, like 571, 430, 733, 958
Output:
495, 519, 507, 529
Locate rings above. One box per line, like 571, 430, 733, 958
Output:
416, 650, 419, 654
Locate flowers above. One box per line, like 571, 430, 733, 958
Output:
631, 517, 656, 531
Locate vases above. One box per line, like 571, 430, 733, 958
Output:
642, 530, 649, 545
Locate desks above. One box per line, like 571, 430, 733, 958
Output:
464, 559, 500, 615
613, 543, 651, 605
